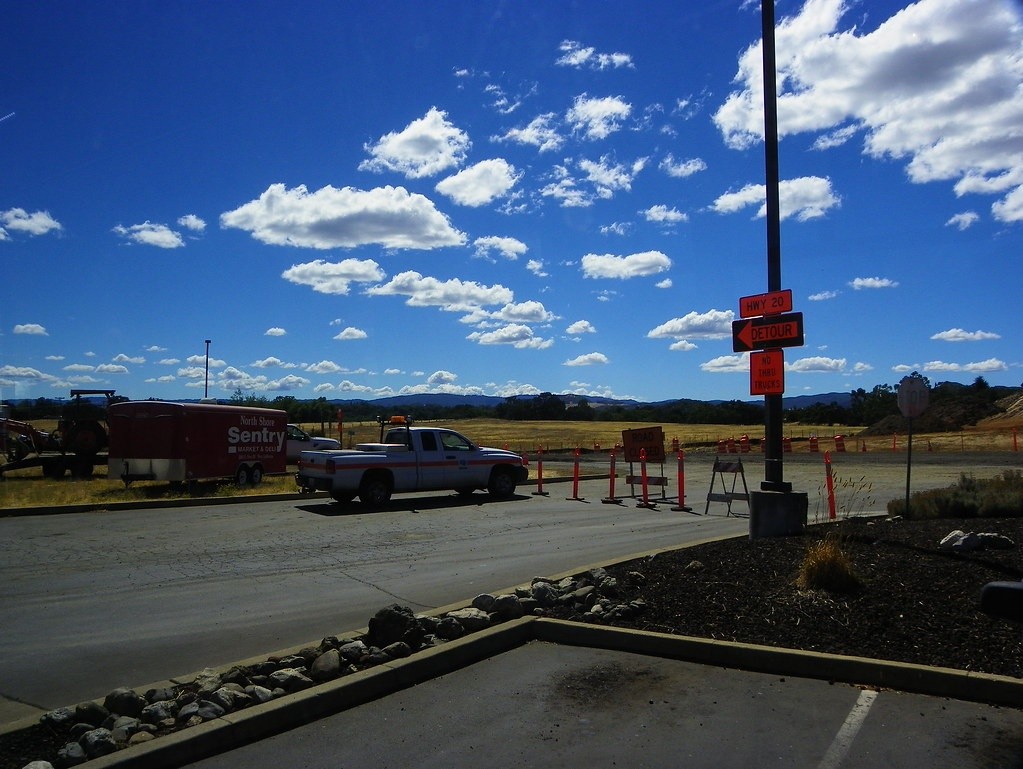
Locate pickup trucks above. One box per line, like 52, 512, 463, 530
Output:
299, 415, 529, 510
283, 423, 341, 461
